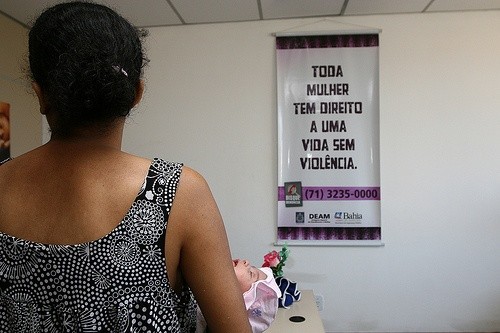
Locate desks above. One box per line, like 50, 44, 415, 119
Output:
258, 290, 326, 333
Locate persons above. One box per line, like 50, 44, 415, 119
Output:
0, 112, 11, 148
0, 0, 254, 332
195, 259, 282, 333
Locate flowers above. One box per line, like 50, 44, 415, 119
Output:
258, 241, 291, 277
